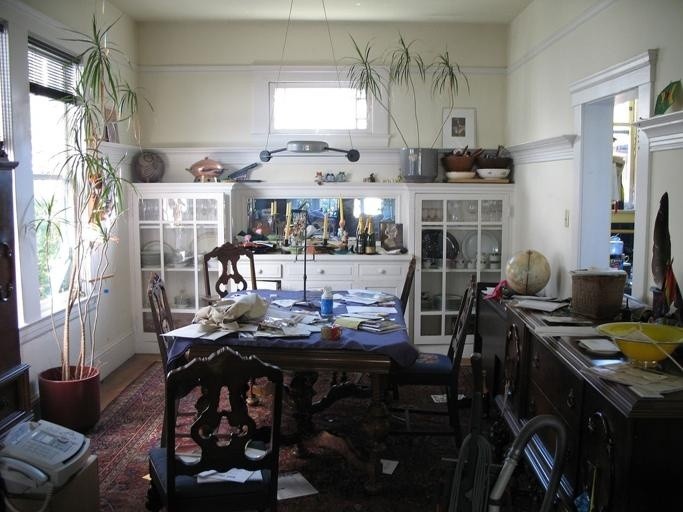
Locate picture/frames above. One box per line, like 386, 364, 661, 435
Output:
441, 107, 478, 150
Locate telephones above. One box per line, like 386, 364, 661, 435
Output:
0, 457, 48, 488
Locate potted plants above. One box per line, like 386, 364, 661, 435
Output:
336, 32, 470, 182
18, 14, 154, 436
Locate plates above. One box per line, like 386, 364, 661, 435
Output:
446, 169, 511, 179
422, 229, 459, 258
141, 232, 218, 262
461, 231, 499, 260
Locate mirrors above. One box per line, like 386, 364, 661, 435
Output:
244, 194, 400, 243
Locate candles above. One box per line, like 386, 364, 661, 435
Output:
274, 201, 276, 214
285, 214, 292, 240
324, 214, 327, 239
271, 201, 273, 216
286, 203, 289, 217
288, 203, 291, 214
340, 198, 345, 223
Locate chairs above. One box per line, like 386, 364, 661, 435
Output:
331, 255, 415, 388
146, 347, 283, 511
147, 272, 245, 449
398, 286, 475, 447
203, 242, 264, 407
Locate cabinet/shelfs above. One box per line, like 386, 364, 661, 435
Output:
407, 184, 515, 357
125, 182, 249, 354
230, 253, 414, 335
474, 282, 683, 512
0, 140, 33, 438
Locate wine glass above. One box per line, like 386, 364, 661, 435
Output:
140, 200, 217, 220
423, 200, 502, 221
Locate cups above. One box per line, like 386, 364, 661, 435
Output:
172, 288, 193, 308
320, 324, 343, 341
423, 253, 501, 270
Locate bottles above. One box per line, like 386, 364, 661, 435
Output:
320, 286, 333, 316
355, 215, 377, 255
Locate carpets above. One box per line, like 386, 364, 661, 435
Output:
89, 362, 489, 512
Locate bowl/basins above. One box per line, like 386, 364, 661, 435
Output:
440, 156, 511, 171
595, 321, 683, 369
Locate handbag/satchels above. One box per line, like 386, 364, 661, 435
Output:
194, 293, 268, 330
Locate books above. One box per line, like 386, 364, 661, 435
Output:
336, 314, 401, 333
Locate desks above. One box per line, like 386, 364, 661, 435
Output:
164, 290, 417, 496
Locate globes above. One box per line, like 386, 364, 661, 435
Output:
505, 249, 551, 297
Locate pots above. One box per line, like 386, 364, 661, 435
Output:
185, 156, 229, 182
